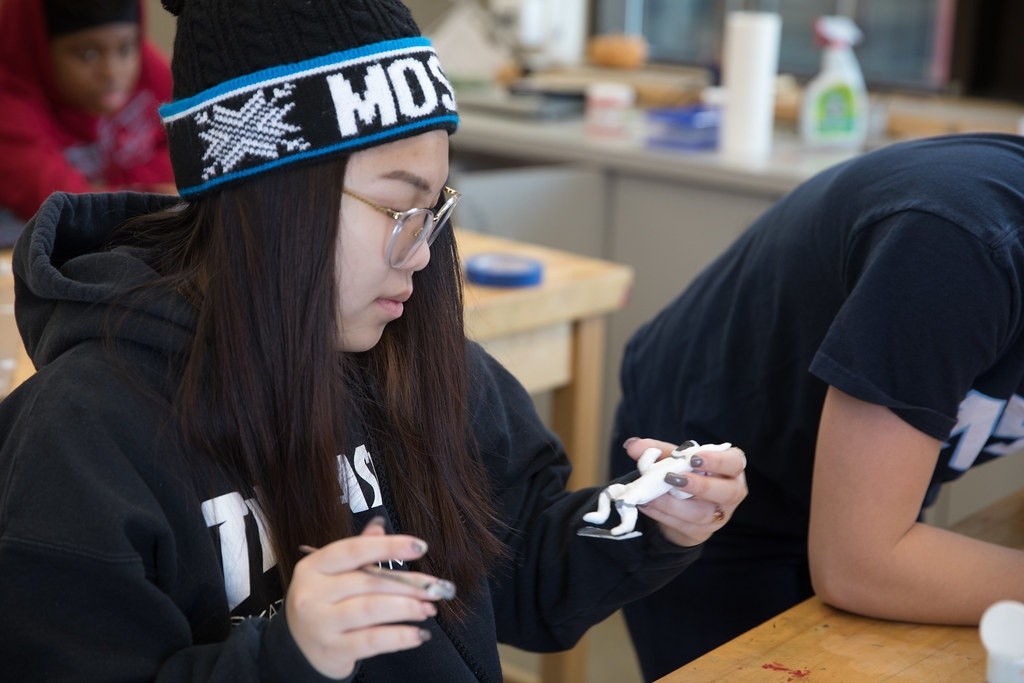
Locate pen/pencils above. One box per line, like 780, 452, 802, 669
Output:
301, 543, 454, 602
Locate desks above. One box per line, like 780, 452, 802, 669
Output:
0, 64, 1024, 683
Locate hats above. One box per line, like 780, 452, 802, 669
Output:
41, 0, 141, 39
157, 0, 462, 201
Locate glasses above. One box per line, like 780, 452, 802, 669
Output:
341, 186, 462, 269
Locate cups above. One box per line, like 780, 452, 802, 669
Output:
590, 84, 633, 134
719, 12, 782, 154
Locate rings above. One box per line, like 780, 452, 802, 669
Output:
712, 503, 725, 524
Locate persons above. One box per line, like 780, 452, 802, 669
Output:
0, 0, 179, 223
0, 0, 752, 683
603, 132, 1024, 683
